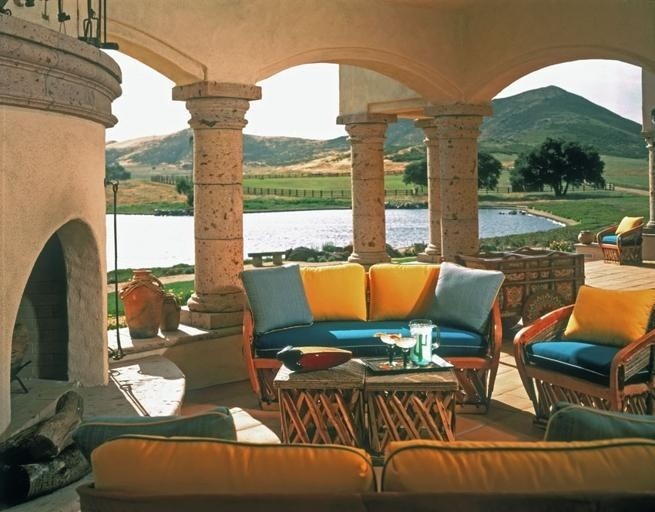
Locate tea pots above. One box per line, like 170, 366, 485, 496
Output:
407, 319, 441, 366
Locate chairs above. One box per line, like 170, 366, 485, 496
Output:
512, 304, 654, 429
595, 218, 645, 266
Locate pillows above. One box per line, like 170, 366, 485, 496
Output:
614, 216, 644, 235
427, 261, 506, 336
68, 406, 238, 466
558, 283, 655, 345
543, 401, 654, 443
238, 264, 313, 335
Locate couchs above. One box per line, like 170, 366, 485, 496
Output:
241, 269, 503, 416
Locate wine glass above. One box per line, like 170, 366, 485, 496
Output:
395, 336, 417, 369
379, 333, 402, 368
